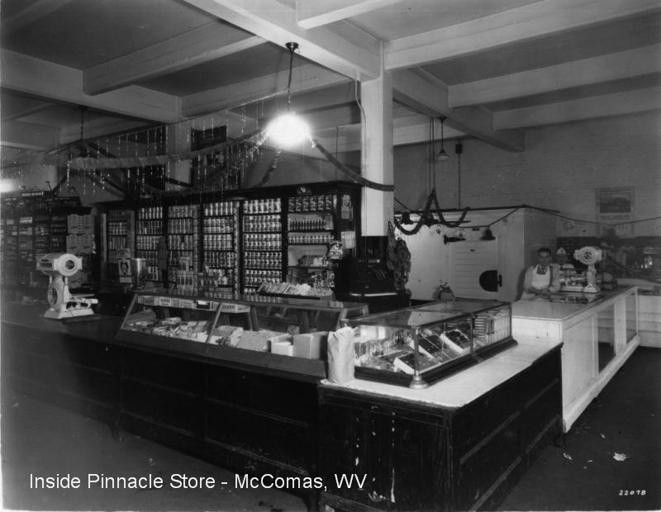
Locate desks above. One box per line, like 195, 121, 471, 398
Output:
509, 283, 641, 434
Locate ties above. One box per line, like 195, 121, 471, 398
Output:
560, 264, 564, 269
541, 266, 545, 273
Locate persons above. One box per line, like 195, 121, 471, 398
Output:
522, 247, 561, 300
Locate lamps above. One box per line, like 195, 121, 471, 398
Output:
262, 41, 311, 152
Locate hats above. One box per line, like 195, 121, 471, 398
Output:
556, 248, 566, 255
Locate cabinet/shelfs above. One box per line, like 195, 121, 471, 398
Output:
98, 199, 135, 283
240, 185, 284, 300
201, 189, 239, 287
339, 297, 518, 389
34, 203, 92, 293
135, 196, 165, 284
163, 194, 202, 287
285, 180, 362, 290
317, 340, 565, 512
1, 212, 33, 293
2, 299, 327, 496
